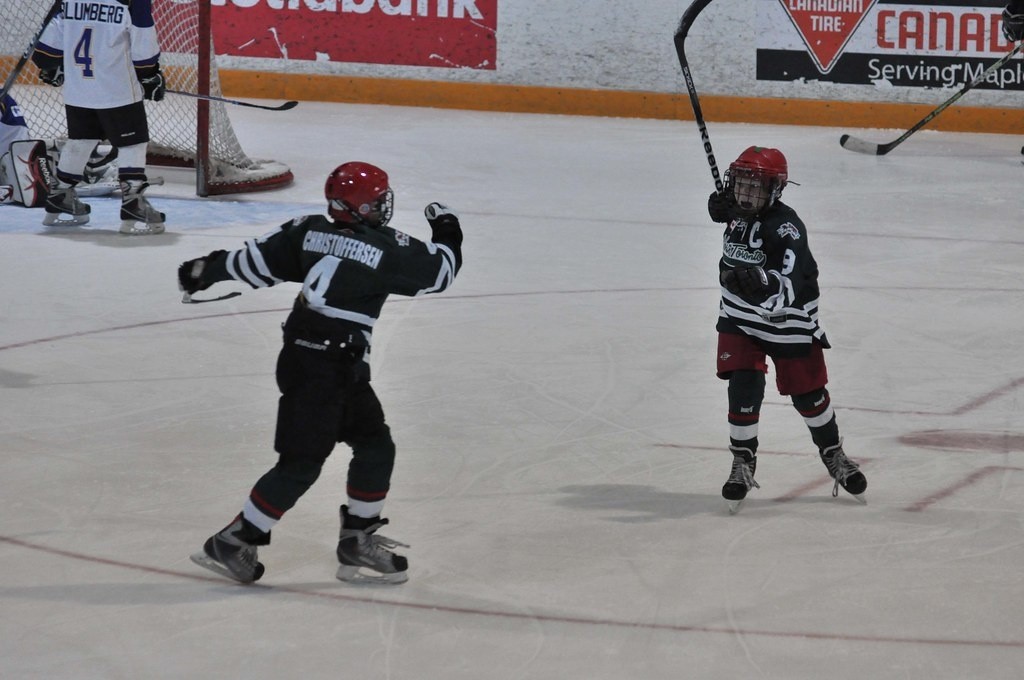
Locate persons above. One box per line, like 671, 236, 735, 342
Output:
1001, 0, 1024, 166
708, 146, 867, 515
31, 0, 166, 235
177, 160, 464, 587
0, 87, 30, 205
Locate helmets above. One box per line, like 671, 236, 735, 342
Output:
324, 161, 393, 229
720, 144, 789, 218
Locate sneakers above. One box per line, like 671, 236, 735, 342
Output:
41, 175, 92, 227
190, 509, 271, 583
333, 504, 410, 585
119, 178, 167, 235
818, 435, 869, 506
721, 444, 760, 515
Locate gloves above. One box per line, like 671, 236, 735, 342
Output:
178, 248, 241, 307
731, 265, 780, 308
37, 62, 64, 87
707, 189, 738, 225
138, 70, 165, 102
424, 201, 459, 225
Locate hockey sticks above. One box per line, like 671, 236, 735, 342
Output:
2, 0, 63, 93
673, 0, 726, 195
841, 42, 1024, 156
182, 289, 242, 303
163, 88, 298, 111
